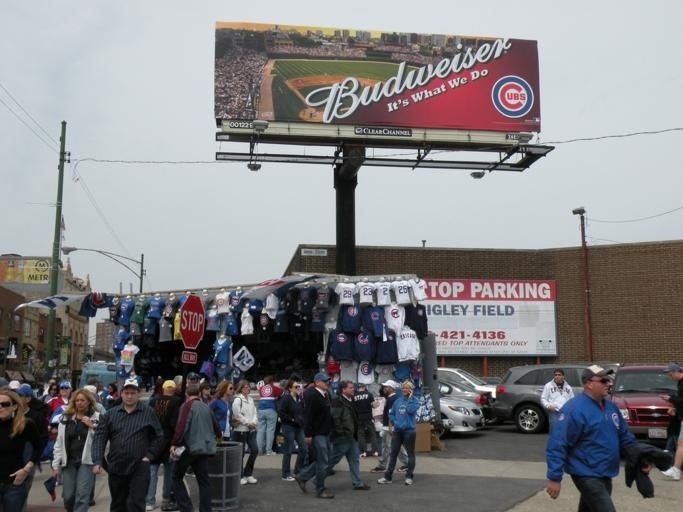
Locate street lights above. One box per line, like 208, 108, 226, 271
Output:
61, 246, 145, 292
571, 207, 595, 363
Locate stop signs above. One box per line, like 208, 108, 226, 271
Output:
178, 295, 206, 351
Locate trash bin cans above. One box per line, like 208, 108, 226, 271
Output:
374, 438, 382, 456
198, 441, 243, 512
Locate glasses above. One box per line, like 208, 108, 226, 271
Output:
587, 378, 610, 383
292, 385, 301, 389
0, 400, 14, 408
229, 387, 233, 390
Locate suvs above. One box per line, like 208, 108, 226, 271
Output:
612, 364, 678, 445
494, 363, 614, 435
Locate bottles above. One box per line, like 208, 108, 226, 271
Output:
4, 260, 16, 280
170, 446, 186, 459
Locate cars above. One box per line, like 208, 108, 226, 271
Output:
437, 366, 504, 439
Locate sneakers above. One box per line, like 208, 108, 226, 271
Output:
370, 466, 388, 473
404, 478, 414, 486
660, 465, 681, 481
351, 483, 372, 491
396, 465, 409, 472
259, 450, 277, 456
240, 476, 248, 485
246, 476, 258, 484
316, 488, 335, 499
88, 498, 95, 507
376, 477, 392, 484
359, 450, 379, 457
294, 473, 307, 494
281, 473, 296, 481
146, 498, 179, 511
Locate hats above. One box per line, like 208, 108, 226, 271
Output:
59, 381, 72, 389
580, 362, 615, 383
119, 378, 140, 391
380, 379, 398, 389
161, 378, 176, 390
8, 380, 33, 397
313, 372, 331, 382
662, 362, 681, 374
186, 371, 198, 380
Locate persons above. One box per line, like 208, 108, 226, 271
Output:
540, 368, 573, 434
255, 374, 421, 498
545, 365, 651, 511
658, 363, 682, 451
1, 371, 259, 511
659, 421, 683, 480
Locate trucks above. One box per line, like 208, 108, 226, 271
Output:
79, 362, 117, 389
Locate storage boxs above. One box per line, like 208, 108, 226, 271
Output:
410, 419, 434, 453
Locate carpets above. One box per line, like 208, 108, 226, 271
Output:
375, 416, 554, 463
158, 461, 551, 512
